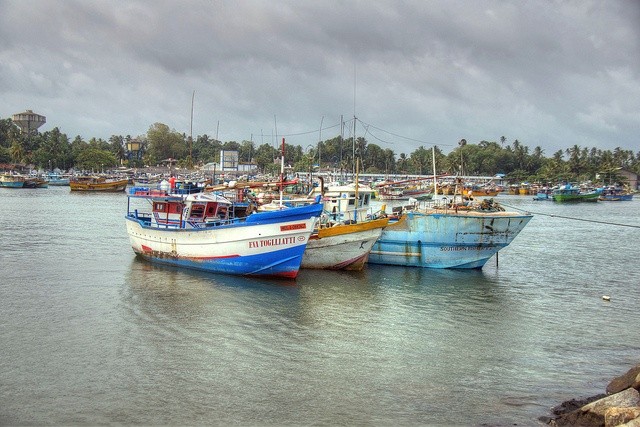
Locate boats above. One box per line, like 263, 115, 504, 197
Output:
131, 172, 150, 186
438, 177, 454, 195
376, 175, 438, 201
124, 136, 324, 280
328, 147, 534, 270
69, 173, 129, 193
552, 187, 599, 203
519, 183, 531, 195
48, 172, 70, 186
507, 183, 520, 195
497, 184, 506, 193
532, 191, 553, 201
259, 156, 388, 273
459, 179, 500, 197
0, 174, 24, 189
599, 188, 632, 202
25, 176, 50, 189
